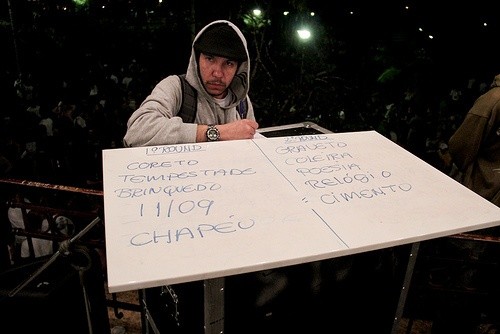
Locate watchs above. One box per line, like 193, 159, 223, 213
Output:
205, 123, 220, 142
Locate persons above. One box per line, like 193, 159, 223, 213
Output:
447, 73, 500, 207
121, 18, 260, 148
0, 53, 152, 267
249, 70, 478, 177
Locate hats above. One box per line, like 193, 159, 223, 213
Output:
194, 22, 247, 64
